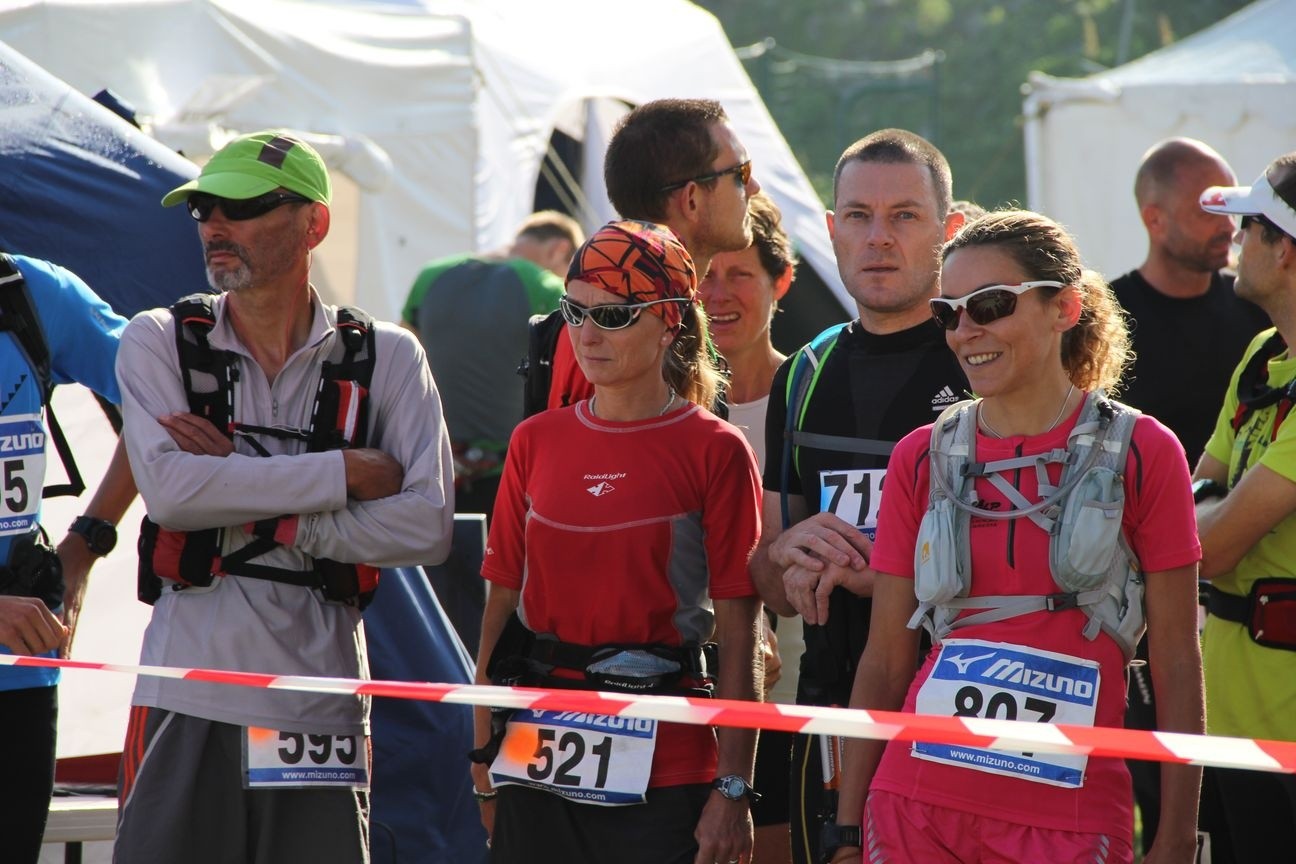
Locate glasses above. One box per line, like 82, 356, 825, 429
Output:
928, 280, 1066, 331
559, 293, 690, 330
1239, 213, 1280, 233
187, 192, 309, 220
657, 160, 752, 194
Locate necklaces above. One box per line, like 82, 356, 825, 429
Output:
591, 382, 675, 423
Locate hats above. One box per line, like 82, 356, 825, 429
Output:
161, 132, 331, 208
1199, 168, 1296, 239
564, 218, 697, 337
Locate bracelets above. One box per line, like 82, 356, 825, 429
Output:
472, 785, 498, 801
819, 812, 862, 862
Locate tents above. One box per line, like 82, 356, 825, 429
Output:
0, 40, 223, 318
1022, 0, 1296, 285
0, 0, 859, 354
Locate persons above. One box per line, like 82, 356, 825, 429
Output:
470, 220, 767, 864
112, 130, 455, 864
397, 208, 585, 663
1107, 137, 1273, 477
1190, 152, 1296, 864
817, 210, 1205, 864
545, 97, 795, 488
748, 128, 978, 863
0, 252, 138, 864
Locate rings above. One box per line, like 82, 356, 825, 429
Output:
728, 859, 739, 864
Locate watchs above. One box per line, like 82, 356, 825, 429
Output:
712, 774, 762, 805
68, 515, 117, 557
1192, 478, 1229, 506
